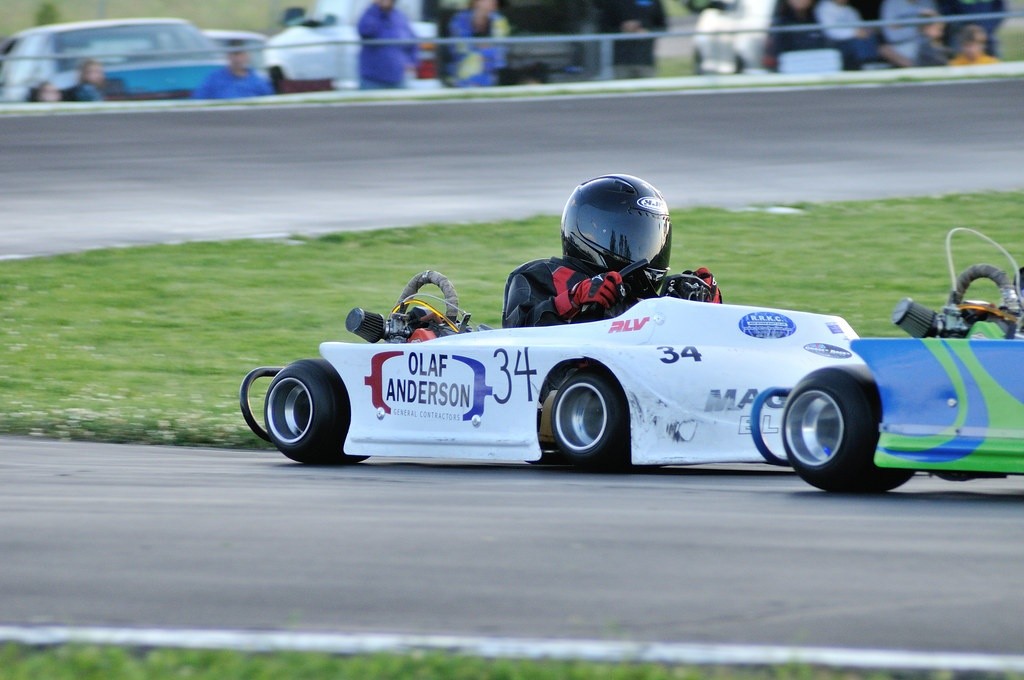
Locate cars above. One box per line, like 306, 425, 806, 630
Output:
692, 0, 784, 75
0, 17, 273, 104
263, 0, 446, 93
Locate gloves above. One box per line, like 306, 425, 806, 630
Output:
570, 271, 624, 312
692, 268, 722, 303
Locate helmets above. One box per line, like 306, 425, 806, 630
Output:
560, 174, 673, 291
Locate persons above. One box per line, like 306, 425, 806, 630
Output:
599, 0, 666, 66
34, 82, 63, 101
66, 60, 104, 101
692, 1, 743, 75
191, 39, 277, 99
444, 0, 511, 86
502, 173, 722, 327
358, 0, 424, 90
763, 0, 1006, 73
